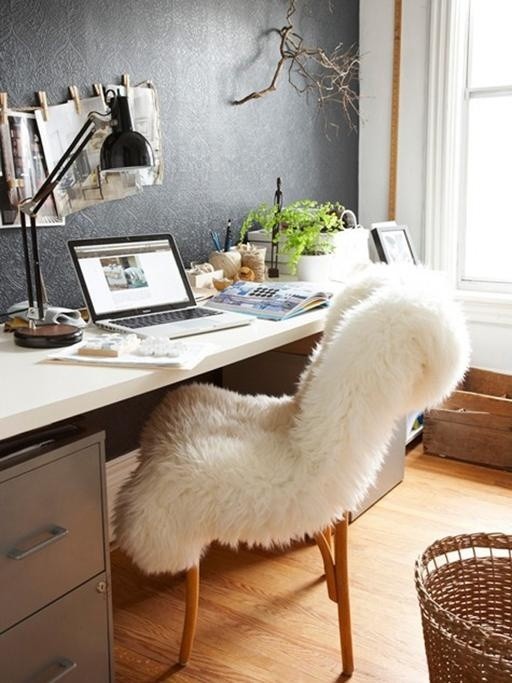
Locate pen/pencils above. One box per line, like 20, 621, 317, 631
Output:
208, 218, 232, 252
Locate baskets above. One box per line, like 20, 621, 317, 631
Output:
411, 531, 512, 683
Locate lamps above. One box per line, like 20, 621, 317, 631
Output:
12, 86, 157, 349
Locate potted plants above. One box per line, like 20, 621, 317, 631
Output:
239, 198, 346, 287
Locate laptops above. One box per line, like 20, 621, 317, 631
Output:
66, 232, 252, 341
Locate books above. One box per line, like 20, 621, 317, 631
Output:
204, 278, 336, 324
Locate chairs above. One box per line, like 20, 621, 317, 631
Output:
111, 261, 474, 676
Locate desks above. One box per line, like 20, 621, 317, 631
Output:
1, 282, 406, 683
